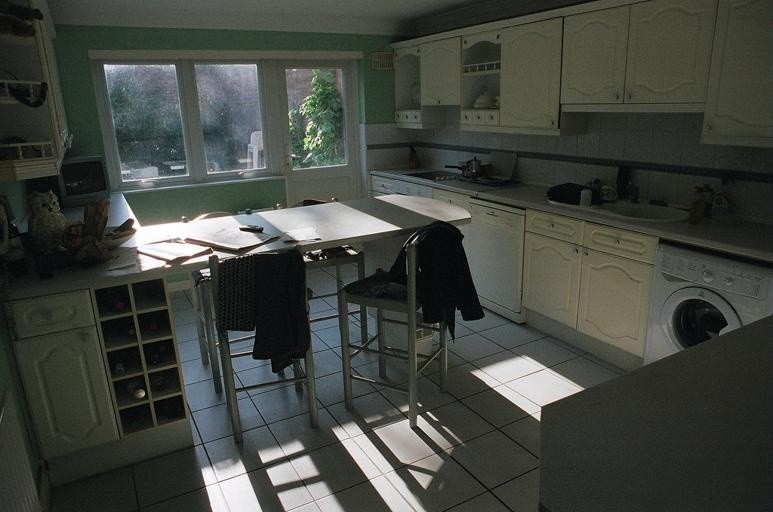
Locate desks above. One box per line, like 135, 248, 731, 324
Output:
5, 195, 471, 366
539, 316, 772, 512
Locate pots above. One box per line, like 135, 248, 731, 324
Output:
444, 156, 484, 179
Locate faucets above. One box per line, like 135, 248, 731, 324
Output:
624, 182, 639, 202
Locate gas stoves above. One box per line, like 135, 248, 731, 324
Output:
402, 170, 463, 182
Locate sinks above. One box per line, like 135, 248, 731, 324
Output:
600, 199, 688, 222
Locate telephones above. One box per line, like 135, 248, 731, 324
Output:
0, 206, 9, 255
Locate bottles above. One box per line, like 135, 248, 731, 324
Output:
410, 77, 421, 108
407, 144, 417, 169
0, 195, 28, 277
112, 294, 167, 400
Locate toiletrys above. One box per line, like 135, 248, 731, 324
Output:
691, 186, 706, 223
711, 172, 734, 223
701, 182, 713, 218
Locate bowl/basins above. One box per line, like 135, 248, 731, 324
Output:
97, 225, 136, 250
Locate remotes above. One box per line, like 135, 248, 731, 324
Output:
240, 225, 263, 233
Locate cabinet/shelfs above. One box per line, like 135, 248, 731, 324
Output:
460, 30, 501, 135
420, 36, 461, 107
0, 0, 73, 181
698, 0, 773, 146
501, 17, 588, 137
389, 43, 442, 130
522, 207, 659, 373
2, 269, 194, 488
560, 0, 720, 113
368, 168, 527, 325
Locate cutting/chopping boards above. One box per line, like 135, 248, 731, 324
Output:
485, 150, 517, 182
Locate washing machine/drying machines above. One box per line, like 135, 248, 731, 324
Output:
643, 243, 773, 369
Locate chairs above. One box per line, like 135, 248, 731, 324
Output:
338, 244, 448, 429
275, 198, 368, 354
209, 244, 319, 444
247, 131, 263, 169
181, 208, 285, 394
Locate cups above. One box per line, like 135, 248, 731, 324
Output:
580, 190, 592, 209
